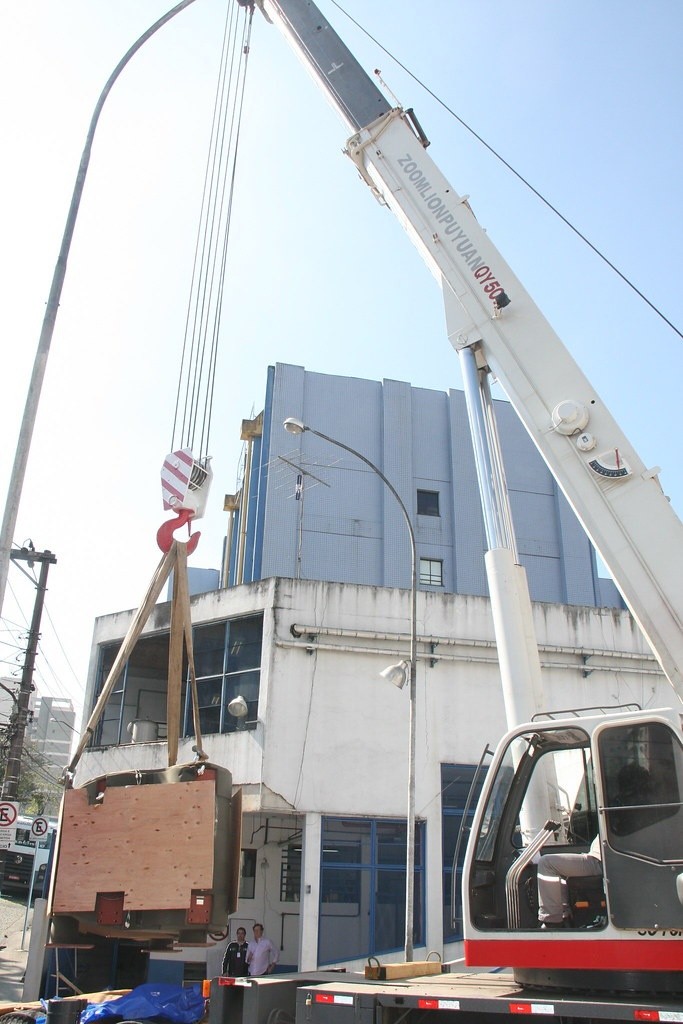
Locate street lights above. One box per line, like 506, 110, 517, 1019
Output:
284, 413, 425, 964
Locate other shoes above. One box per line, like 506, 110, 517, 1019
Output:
540, 922, 566, 929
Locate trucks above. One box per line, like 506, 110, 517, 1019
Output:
0, 816, 58, 899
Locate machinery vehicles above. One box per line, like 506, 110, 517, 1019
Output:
55, 3, 683, 1023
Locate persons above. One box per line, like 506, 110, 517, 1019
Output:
245, 924, 279, 976
537, 763, 650, 929
222, 927, 249, 977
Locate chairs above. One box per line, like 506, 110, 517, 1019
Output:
566, 763, 657, 896
